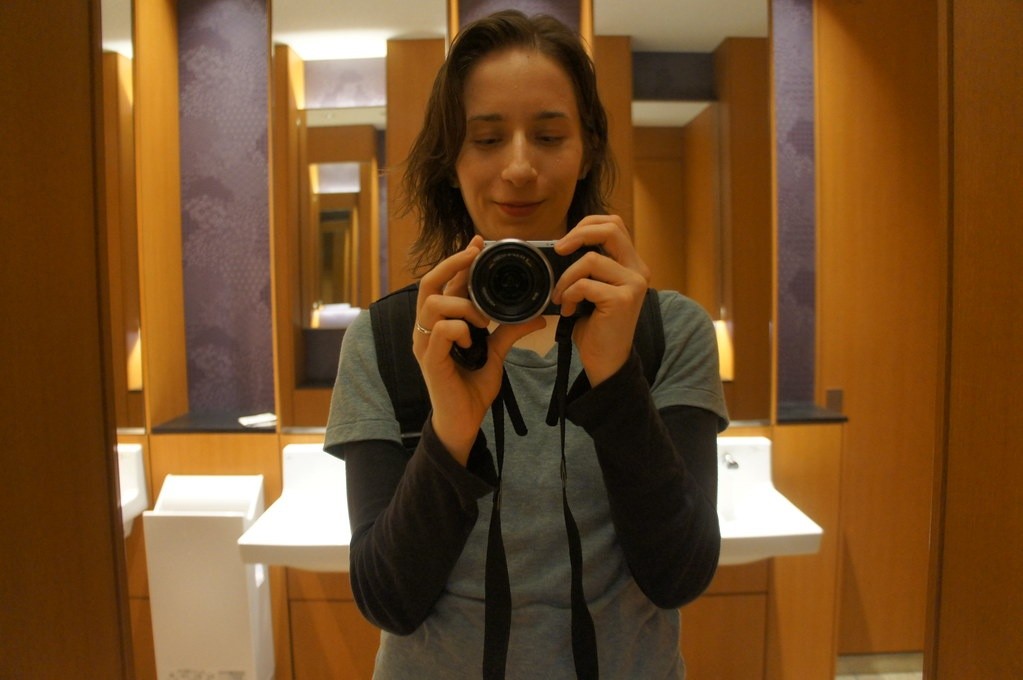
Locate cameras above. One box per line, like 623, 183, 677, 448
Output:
466, 237, 600, 325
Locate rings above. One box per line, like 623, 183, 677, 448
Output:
417, 324, 431, 334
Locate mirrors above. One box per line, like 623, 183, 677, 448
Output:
100, 0, 149, 436
266, 0, 775, 438
85, 0, 958, 680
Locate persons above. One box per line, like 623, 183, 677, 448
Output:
324, 9, 731, 680
